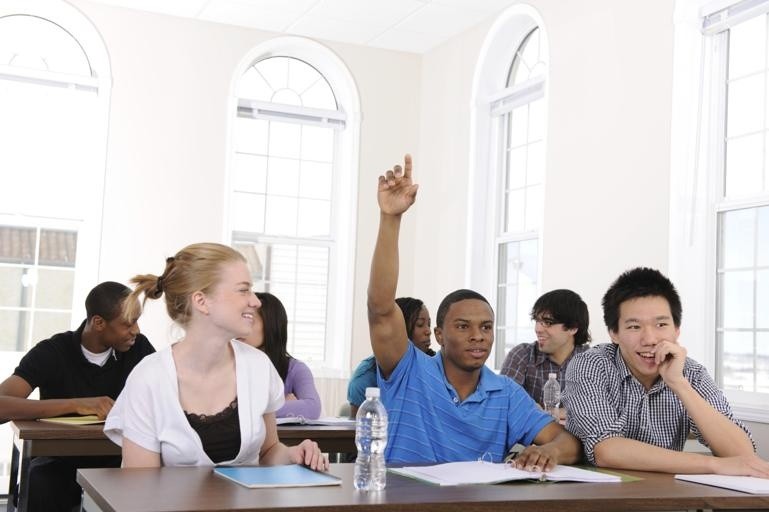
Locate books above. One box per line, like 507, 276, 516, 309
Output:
269, 415, 359, 428
672, 473, 769, 496
209, 464, 344, 489
33, 415, 109, 428
385, 457, 646, 488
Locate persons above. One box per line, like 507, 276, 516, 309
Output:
364, 148, 587, 473
337, 298, 437, 462
500, 288, 592, 431
562, 265, 769, 477
100, 239, 337, 477
233, 291, 332, 466
0, 281, 159, 509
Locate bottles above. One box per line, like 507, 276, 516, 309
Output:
541, 373, 563, 416
352, 386, 390, 491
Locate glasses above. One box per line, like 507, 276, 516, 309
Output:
533, 317, 563, 329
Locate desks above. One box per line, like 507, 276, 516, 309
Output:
72, 452, 768, 511
5, 414, 356, 511
